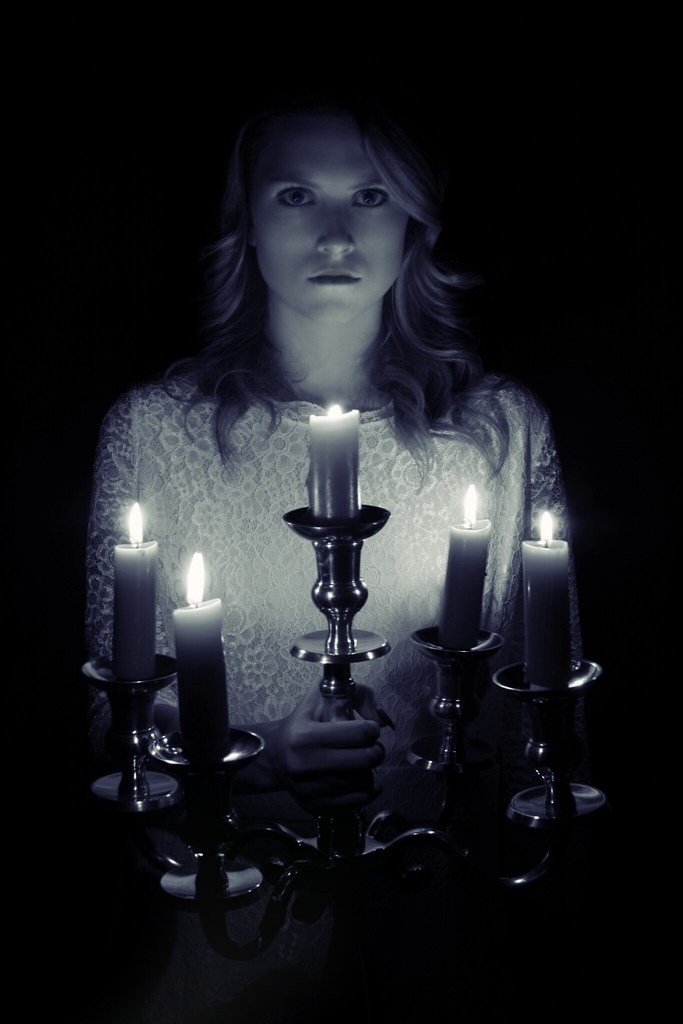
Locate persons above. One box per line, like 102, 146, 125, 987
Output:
82, 109, 585, 1024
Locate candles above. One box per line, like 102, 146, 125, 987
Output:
303, 402, 363, 517
112, 498, 157, 682
520, 511, 572, 682
172, 552, 230, 756
437, 486, 493, 645
